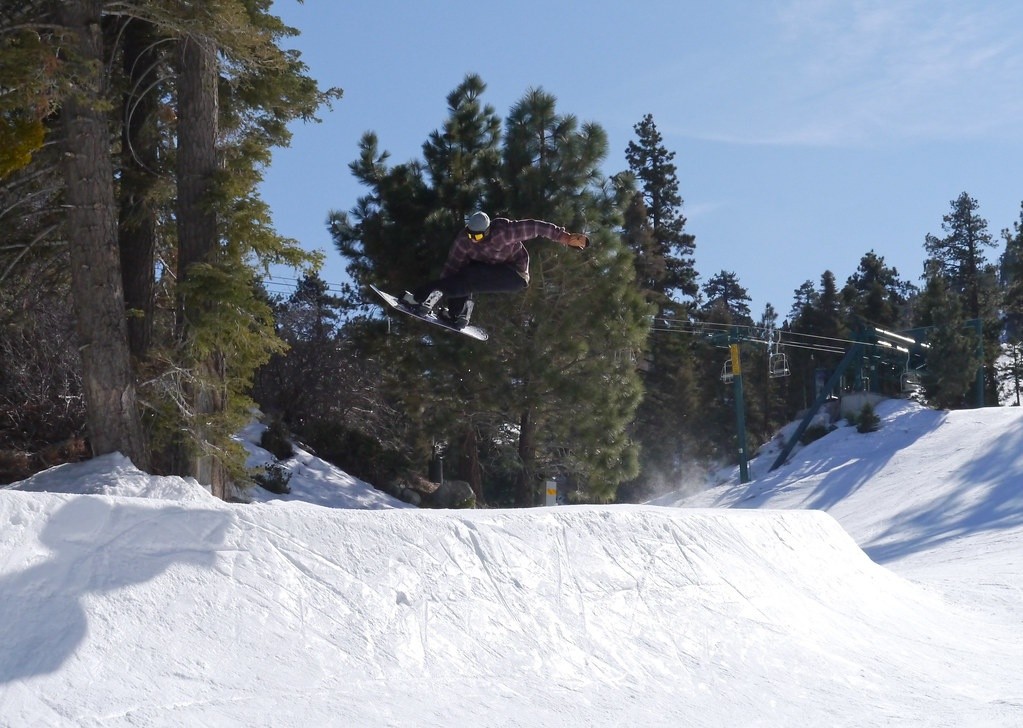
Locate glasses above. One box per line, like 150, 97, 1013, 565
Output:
465, 230, 485, 241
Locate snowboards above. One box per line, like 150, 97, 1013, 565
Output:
370, 284, 489, 342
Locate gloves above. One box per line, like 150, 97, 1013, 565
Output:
561, 233, 591, 251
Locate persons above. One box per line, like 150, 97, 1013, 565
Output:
397, 211, 587, 329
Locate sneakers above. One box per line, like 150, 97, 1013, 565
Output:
437, 300, 475, 329
399, 290, 443, 315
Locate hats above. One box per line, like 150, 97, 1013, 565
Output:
468, 210, 490, 231
